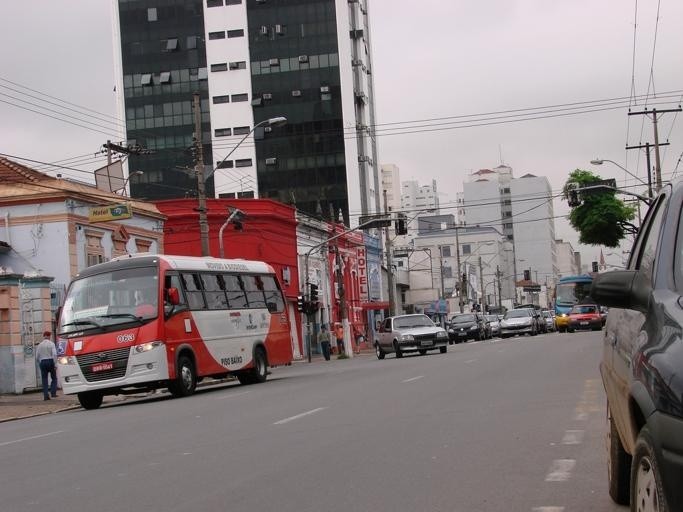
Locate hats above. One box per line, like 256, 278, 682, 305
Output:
43, 332, 51, 336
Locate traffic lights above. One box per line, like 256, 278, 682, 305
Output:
297, 294, 304, 312
311, 284, 319, 313
523, 270, 531, 281
591, 261, 599, 273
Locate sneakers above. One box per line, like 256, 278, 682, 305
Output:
44, 396, 50, 400
51, 392, 58, 397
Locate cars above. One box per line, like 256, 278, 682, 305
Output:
589, 173, 682, 512
449, 309, 554, 344
373, 312, 449, 359
568, 303, 602, 333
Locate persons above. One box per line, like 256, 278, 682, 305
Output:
319, 325, 331, 361
354, 324, 366, 354
332, 325, 345, 355
35, 332, 60, 401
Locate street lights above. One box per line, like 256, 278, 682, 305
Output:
589, 159, 657, 191
456, 243, 527, 311
197, 115, 287, 253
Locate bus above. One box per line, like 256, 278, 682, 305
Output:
56, 252, 293, 411
553, 275, 603, 332
